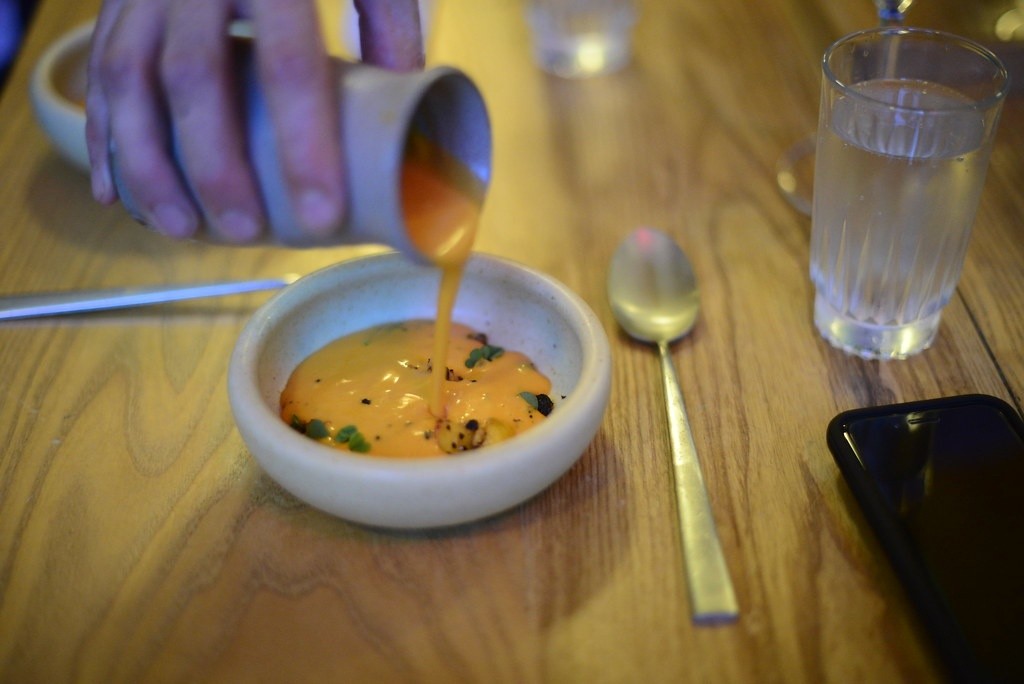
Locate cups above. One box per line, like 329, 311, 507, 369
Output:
812, 27, 1010, 360
524, 0, 636, 80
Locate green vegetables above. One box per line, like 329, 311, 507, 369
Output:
289, 331, 540, 455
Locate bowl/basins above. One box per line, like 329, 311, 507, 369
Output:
226, 251, 611, 531
32, 21, 256, 159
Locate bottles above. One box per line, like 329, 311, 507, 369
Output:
110, 52, 493, 269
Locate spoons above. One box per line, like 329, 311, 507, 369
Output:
607, 230, 737, 623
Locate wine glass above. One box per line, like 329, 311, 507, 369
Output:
774, 0, 912, 218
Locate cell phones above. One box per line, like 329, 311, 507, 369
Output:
827, 393, 1024, 683
